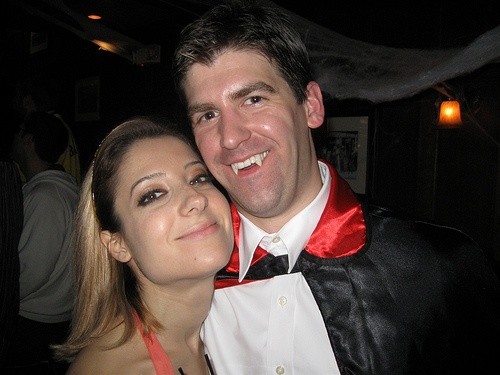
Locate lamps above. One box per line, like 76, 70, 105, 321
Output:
437, 98, 464, 129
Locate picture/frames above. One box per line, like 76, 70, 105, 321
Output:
74, 75, 100, 122
310, 116, 369, 194
30, 32, 49, 54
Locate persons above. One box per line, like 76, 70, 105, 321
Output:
176, 1, 499, 375
51, 118, 235, 374
1, 79, 82, 374
